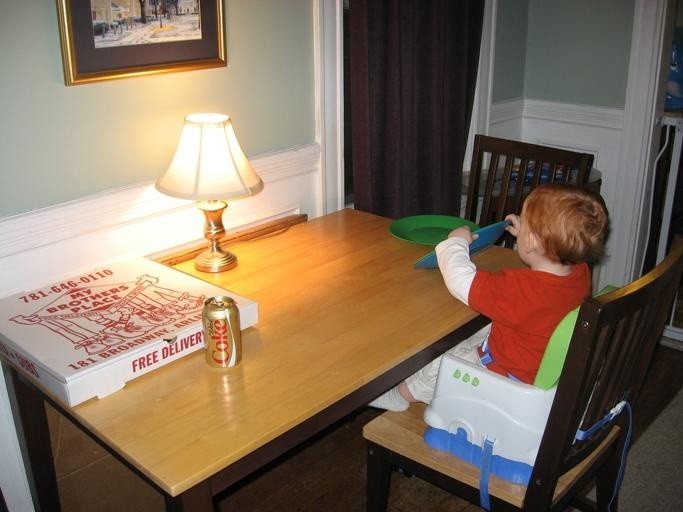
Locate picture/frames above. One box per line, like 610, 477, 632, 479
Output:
55, 0, 228, 87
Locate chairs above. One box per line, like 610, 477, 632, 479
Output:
359, 234, 683, 512
464, 134, 594, 249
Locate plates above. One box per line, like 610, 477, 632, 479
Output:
412, 220, 511, 270
386, 214, 480, 248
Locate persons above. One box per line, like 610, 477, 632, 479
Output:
363, 182, 609, 411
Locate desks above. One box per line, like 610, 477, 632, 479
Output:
655, 111, 682, 266
0, 208, 530, 512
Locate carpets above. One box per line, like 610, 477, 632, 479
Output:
568, 386, 682, 512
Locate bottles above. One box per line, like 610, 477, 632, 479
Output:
509, 170, 563, 185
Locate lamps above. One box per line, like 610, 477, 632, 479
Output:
156, 112, 264, 274
